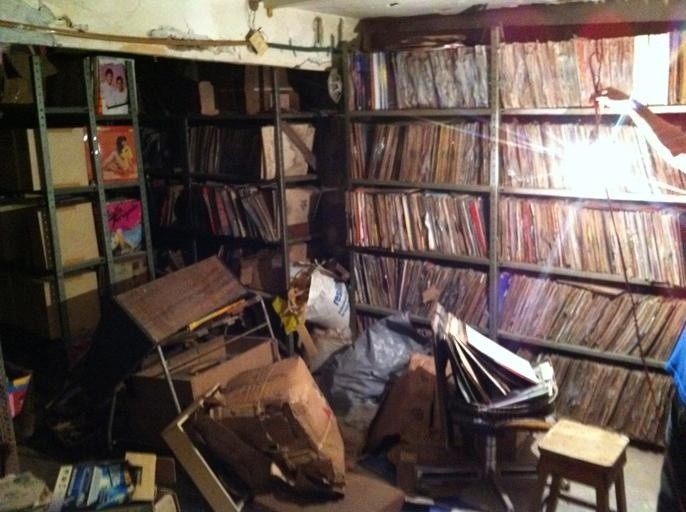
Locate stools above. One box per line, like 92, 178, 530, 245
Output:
254, 474, 405, 511
531, 419, 631, 512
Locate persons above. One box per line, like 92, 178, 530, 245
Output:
589, 85, 686, 174
102, 134, 133, 175
108, 75, 128, 105
657, 322, 686, 509
99, 66, 116, 93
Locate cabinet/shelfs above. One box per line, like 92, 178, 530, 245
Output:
339, 27, 685, 452
2, 27, 339, 452
